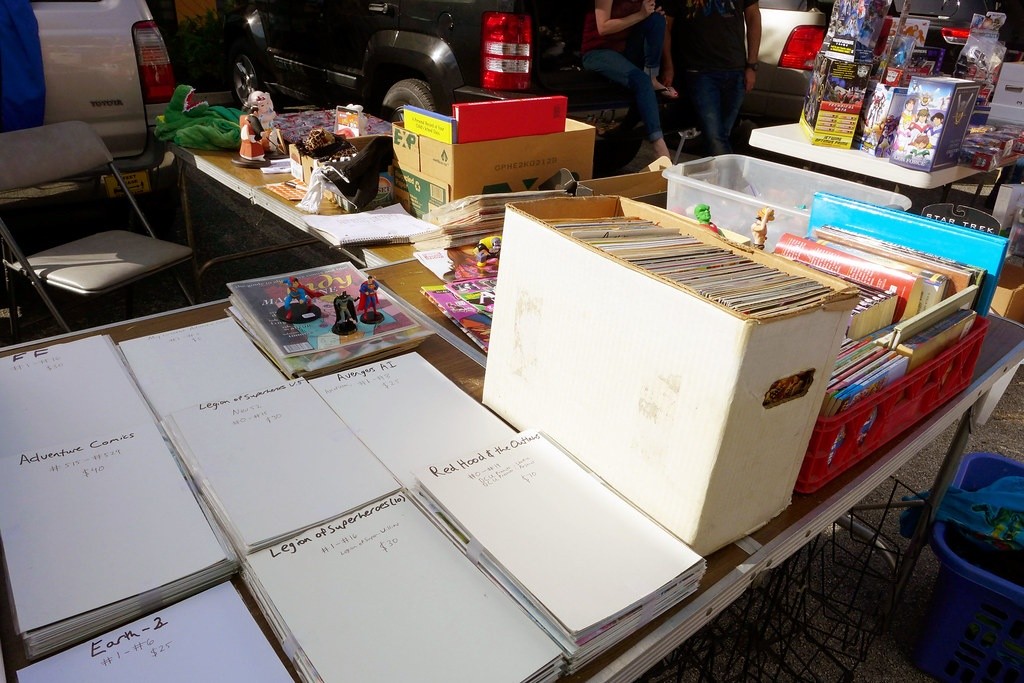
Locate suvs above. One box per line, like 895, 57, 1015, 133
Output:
220, 0, 644, 179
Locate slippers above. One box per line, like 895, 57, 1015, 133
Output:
653, 85, 679, 99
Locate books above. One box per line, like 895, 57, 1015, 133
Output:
225, 260, 436, 380
0, 317, 705, 682
407, 189, 569, 352
400, 89, 568, 144
302, 202, 438, 249
544, 192, 1010, 467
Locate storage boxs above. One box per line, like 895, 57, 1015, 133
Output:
897, 452, 1024, 683
662, 153, 912, 265
794, 313, 995, 493
481, 194, 862, 558
991, 184, 1024, 237
289, 132, 393, 214
392, 119, 597, 221
799, 0, 1024, 174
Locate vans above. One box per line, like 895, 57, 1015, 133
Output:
0, 0, 180, 234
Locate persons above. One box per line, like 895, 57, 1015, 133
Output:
336, 291, 360, 325
249, 106, 275, 155
751, 205, 775, 249
248, 91, 277, 131
360, 276, 379, 320
274, 276, 311, 320
581, 0, 762, 162
695, 204, 726, 239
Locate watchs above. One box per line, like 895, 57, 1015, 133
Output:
747, 63, 759, 71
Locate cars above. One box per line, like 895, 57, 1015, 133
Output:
659, 1, 834, 123
887, 0, 1024, 74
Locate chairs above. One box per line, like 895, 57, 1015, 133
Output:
0, 120, 196, 334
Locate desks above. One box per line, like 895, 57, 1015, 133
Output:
0, 254, 1024, 683
171, 142, 443, 270
749, 123, 1024, 211
